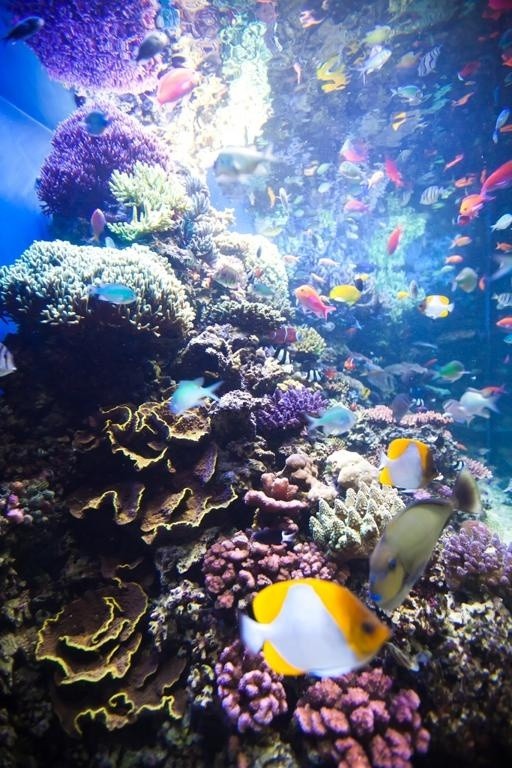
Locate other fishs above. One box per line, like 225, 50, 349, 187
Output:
215, 0, 512, 437
